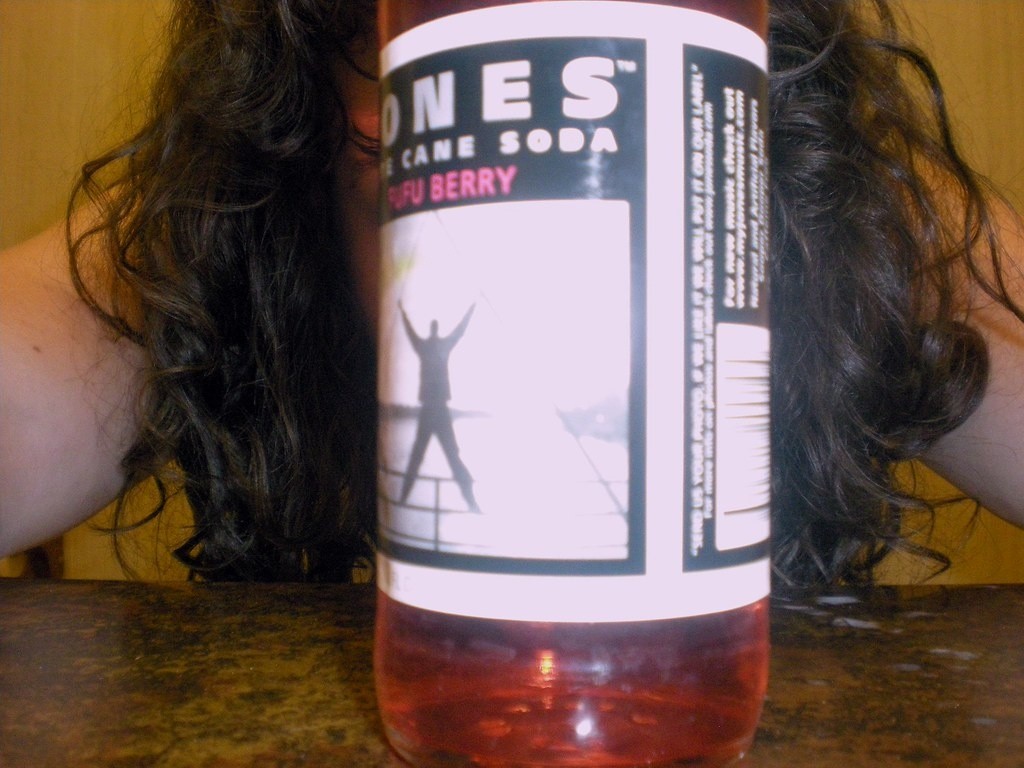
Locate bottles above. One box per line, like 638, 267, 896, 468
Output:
378, 1, 771, 768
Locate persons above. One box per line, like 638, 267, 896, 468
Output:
0, 0, 1024, 582
